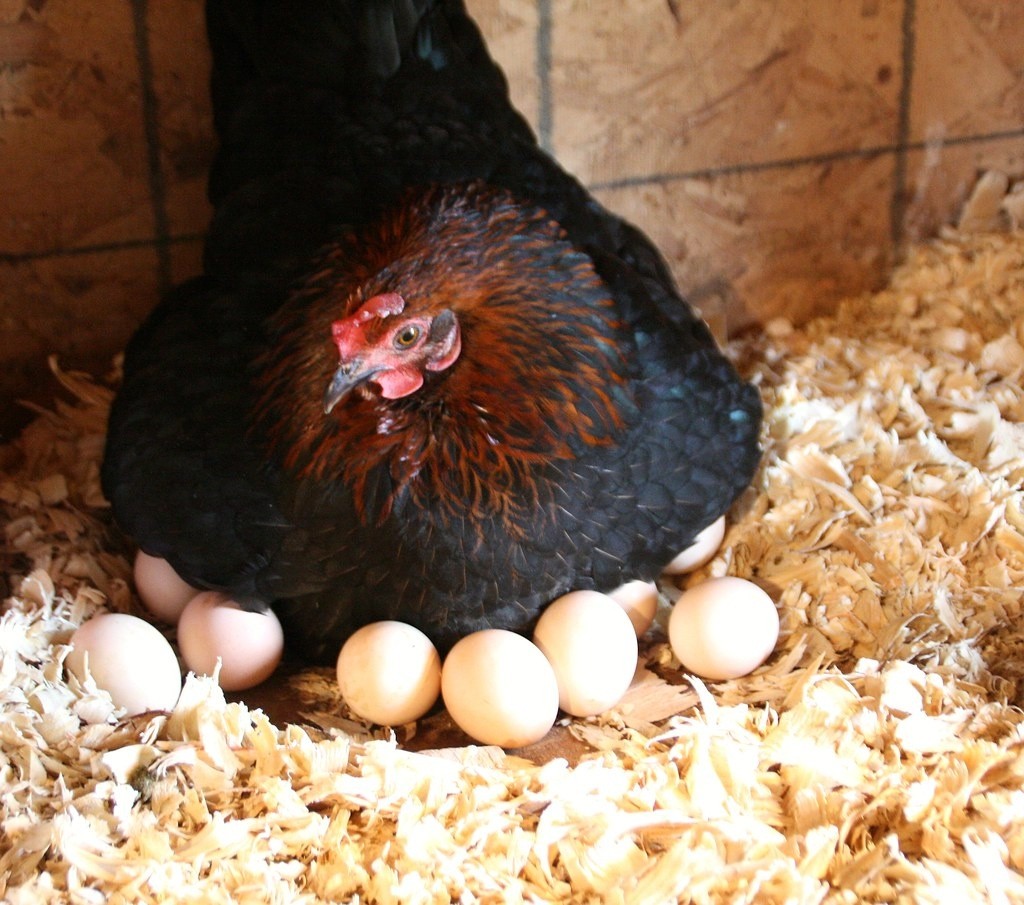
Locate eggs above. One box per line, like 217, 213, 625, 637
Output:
63, 512, 780, 749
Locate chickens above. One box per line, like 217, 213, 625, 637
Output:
95, 0, 769, 671
